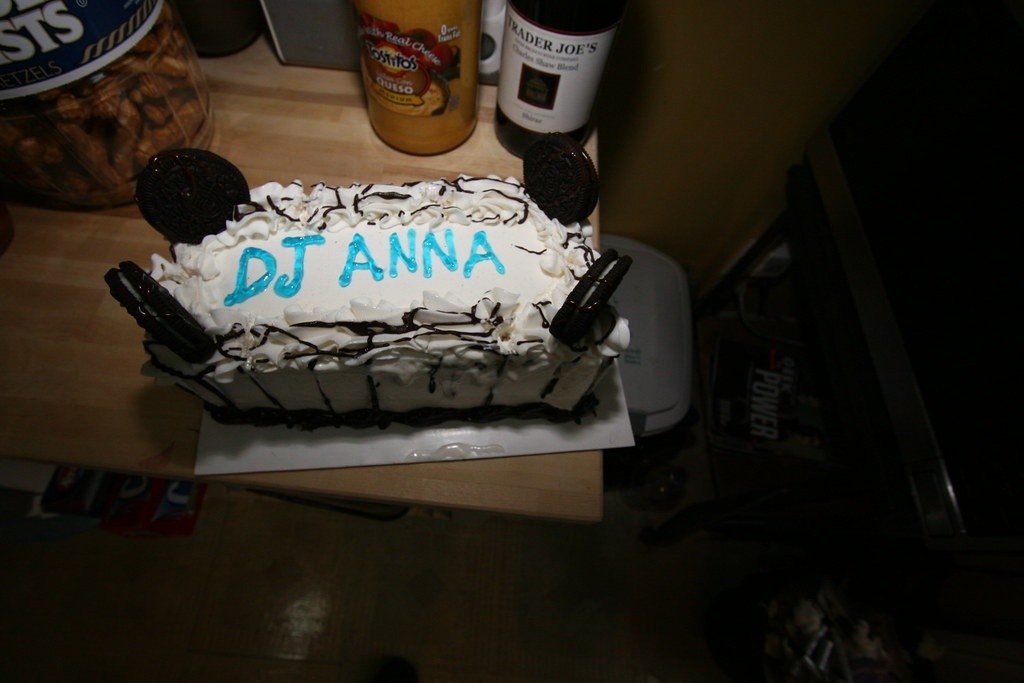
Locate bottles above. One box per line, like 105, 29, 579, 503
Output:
360, 0, 493, 156
491, 0, 639, 163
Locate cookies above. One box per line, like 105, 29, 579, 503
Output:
0, 0, 209, 206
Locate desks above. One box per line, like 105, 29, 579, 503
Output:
0, 34, 607, 528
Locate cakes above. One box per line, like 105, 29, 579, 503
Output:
104, 130, 634, 430
